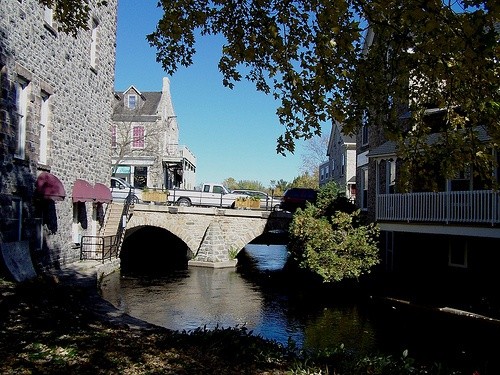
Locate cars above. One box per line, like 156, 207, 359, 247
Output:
111, 177, 148, 204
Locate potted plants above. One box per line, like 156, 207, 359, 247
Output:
141, 189, 170, 202
234, 195, 260, 210
35, 274, 73, 328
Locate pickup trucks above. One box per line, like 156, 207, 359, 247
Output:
167, 183, 254, 210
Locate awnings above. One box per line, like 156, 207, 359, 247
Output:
95, 183, 113, 204
37, 171, 66, 201
73, 179, 96, 203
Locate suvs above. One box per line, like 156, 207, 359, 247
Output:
281, 187, 332, 215
228, 189, 287, 212
